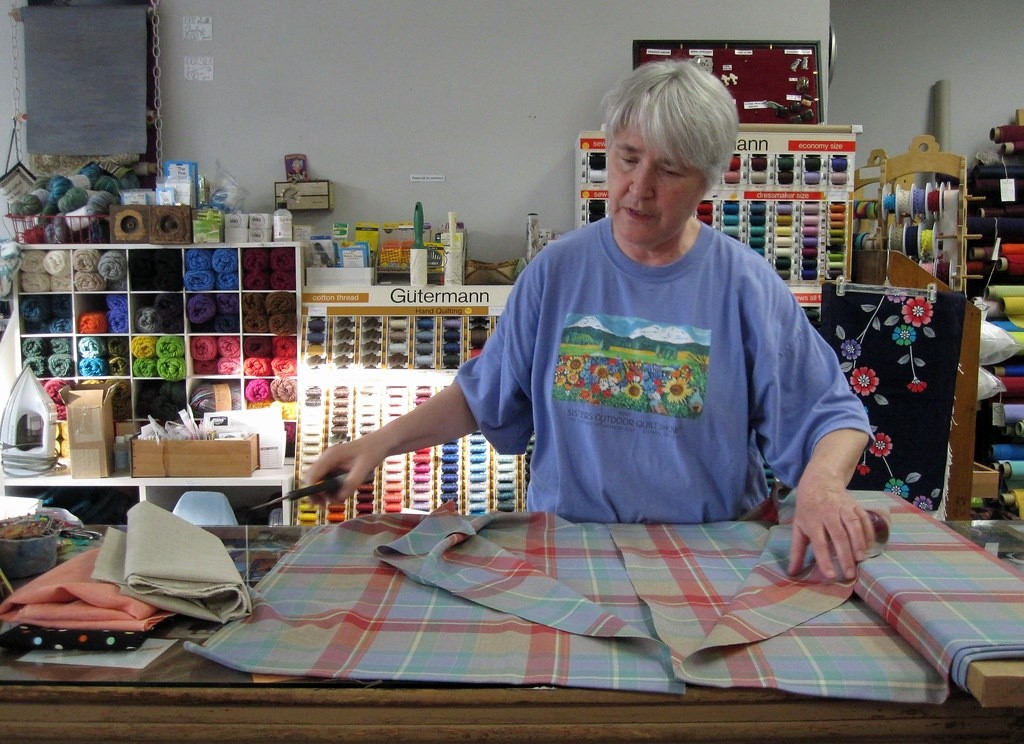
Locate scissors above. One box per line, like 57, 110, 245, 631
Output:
250, 471, 374, 510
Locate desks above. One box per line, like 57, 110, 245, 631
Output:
0, 520, 1023, 744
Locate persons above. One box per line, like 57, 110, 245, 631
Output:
304, 59, 877, 582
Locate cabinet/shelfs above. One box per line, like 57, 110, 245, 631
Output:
852, 249, 998, 523
13, 240, 309, 465
274, 181, 335, 210
2, 465, 294, 525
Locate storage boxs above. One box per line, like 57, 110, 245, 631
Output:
306, 264, 376, 286
129, 431, 261, 478
58, 380, 119, 479
